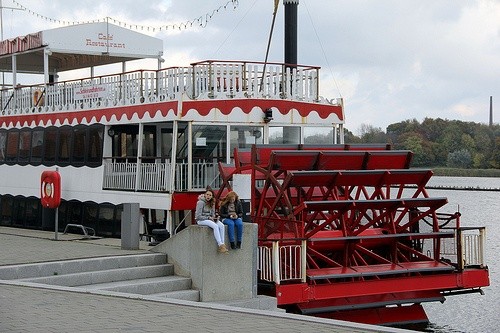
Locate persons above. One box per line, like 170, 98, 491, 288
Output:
194, 185, 229, 253
219, 190, 244, 250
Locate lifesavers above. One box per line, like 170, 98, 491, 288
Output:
32, 89, 44, 109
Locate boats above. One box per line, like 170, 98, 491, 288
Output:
1, 0, 347, 249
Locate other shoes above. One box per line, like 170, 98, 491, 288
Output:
235, 241, 242, 249
218, 246, 226, 253
230, 242, 236, 249
222, 244, 229, 252
